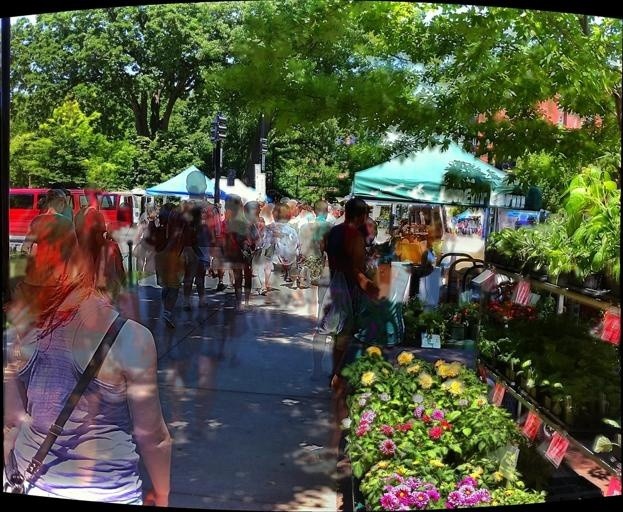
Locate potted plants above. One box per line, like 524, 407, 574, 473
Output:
476, 312, 621, 463
485, 163, 622, 298
401, 294, 476, 348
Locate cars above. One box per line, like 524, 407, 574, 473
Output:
10, 187, 70, 255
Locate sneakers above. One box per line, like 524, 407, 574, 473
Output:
161, 312, 176, 329
259, 288, 267, 295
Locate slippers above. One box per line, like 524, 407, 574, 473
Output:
235, 305, 253, 314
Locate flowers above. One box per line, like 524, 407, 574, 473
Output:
336, 345, 550, 511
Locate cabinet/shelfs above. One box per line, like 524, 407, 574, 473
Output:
475, 263, 622, 482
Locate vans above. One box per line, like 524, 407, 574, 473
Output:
59, 187, 155, 242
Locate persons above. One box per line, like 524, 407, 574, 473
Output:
2, 188, 171, 508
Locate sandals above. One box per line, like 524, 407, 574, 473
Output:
217, 281, 224, 290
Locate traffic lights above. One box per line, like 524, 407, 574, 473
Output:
262, 137, 269, 154
218, 116, 227, 139
209, 119, 217, 143
